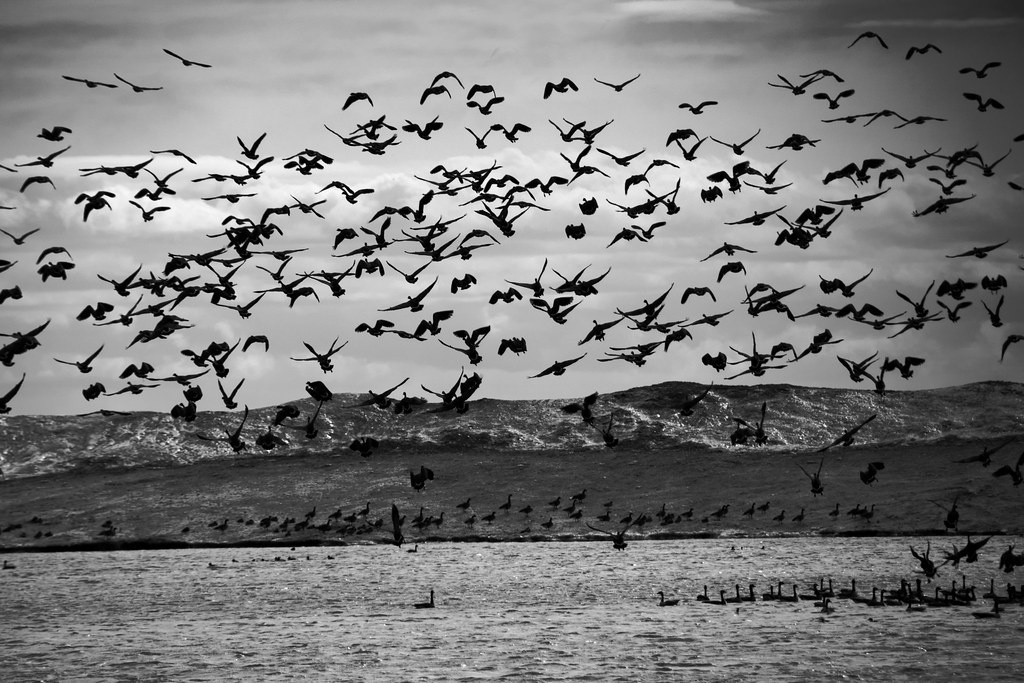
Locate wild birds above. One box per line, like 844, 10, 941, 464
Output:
0, 31, 1024, 622
